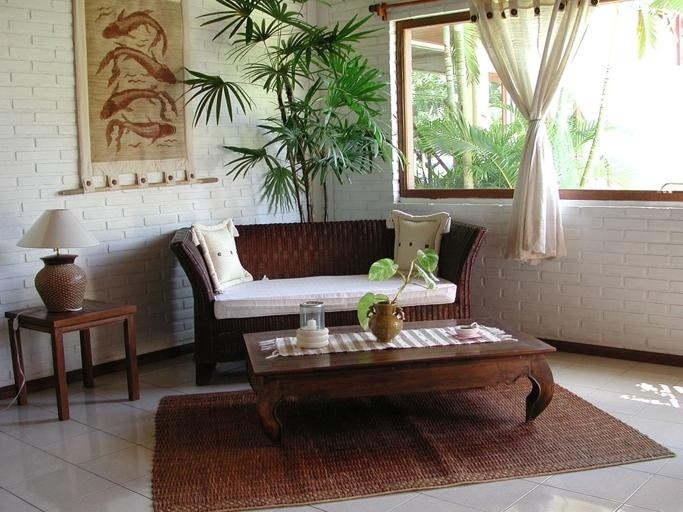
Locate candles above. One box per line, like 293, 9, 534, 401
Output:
307, 313, 316, 330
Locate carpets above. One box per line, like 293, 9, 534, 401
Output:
152, 383, 676, 512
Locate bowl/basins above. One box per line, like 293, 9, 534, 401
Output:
454, 323, 480, 337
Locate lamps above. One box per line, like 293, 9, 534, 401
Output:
16, 209, 101, 313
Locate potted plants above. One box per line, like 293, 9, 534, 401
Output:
358, 249, 439, 343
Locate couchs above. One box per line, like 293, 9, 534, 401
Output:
170, 219, 490, 385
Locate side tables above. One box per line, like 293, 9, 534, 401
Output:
5, 300, 140, 421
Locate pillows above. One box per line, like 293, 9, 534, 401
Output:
392, 209, 452, 277
189, 220, 253, 293
